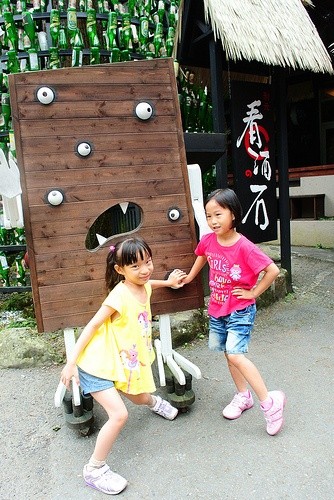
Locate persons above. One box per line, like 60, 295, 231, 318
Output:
61, 238, 187, 494
177, 187, 286, 436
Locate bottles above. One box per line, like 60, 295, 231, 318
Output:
0, 0, 213, 161
0, 195, 32, 287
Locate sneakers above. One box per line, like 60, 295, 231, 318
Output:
260, 390, 285, 435
148, 396, 179, 420
83, 463, 127, 495
222, 389, 254, 419
